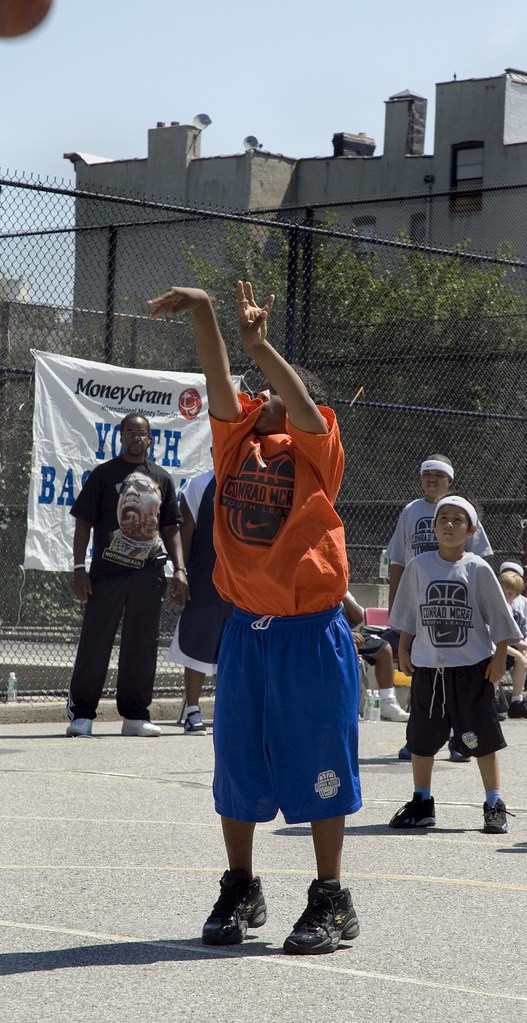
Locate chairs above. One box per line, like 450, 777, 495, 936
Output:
364, 607, 389, 625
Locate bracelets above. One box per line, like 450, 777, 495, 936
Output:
74, 564, 85, 569
175, 566, 188, 575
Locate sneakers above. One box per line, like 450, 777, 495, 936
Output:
398, 746, 411, 760
380, 697, 410, 722
184, 711, 207, 736
482, 798, 516, 833
202, 870, 267, 945
508, 700, 527, 718
284, 879, 360, 955
66, 718, 92, 737
448, 742, 471, 762
389, 792, 436, 828
122, 718, 162, 737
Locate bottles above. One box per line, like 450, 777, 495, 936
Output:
363, 689, 373, 722
8, 672, 17, 704
370, 690, 381, 723
379, 549, 390, 578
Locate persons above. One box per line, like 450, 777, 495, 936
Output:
387, 495, 524, 833
66, 413, 189, 736
382, 455, 493, 762
340, 589, 410, 722
486, 560, 527, 718
166, 443, 235, 736
150, 281, 362, 955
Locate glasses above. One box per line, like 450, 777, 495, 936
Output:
121, 431, 149, 438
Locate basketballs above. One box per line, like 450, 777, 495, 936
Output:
0, 0, 53, 37
